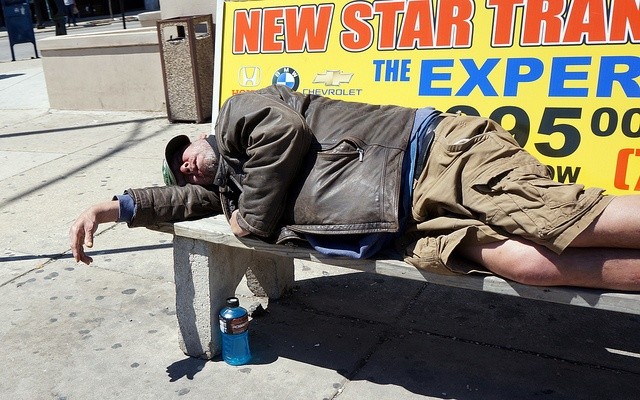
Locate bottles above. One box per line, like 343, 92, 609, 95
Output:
217, 296, 252, 366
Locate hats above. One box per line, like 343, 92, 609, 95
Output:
163, 135, 191, 186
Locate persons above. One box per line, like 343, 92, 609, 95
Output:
69, 84, 637, 293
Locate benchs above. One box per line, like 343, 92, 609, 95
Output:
147, 214, 640, 360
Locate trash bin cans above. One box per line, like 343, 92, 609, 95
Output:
156, 13, 214, 124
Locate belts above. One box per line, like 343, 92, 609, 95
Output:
414, 116, 446, 180
